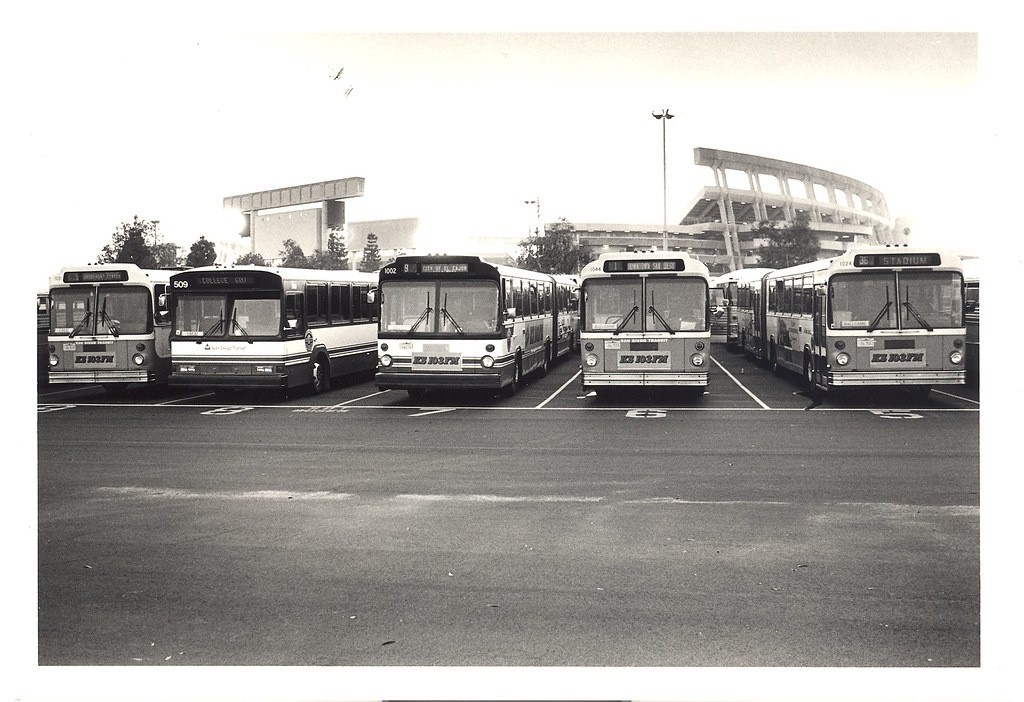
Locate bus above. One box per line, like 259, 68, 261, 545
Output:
159, 264, 431, 396
709, 243, 966, 400
572, 244, 712, 410
37, 263, 278, 394
366, 256, 583, 398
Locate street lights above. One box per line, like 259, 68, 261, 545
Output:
651, 108, 674, 269
150, 220, 160, 245
